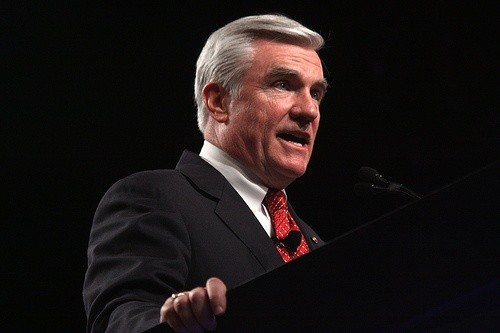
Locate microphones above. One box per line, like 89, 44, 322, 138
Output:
282, 230, 302, 251
358, 166, 420, 200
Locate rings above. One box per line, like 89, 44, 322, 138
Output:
172, 292, 185, 301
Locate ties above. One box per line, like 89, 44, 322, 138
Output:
262, 187, 311, 263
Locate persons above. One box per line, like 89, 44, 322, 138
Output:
83, 14, 329, 333
